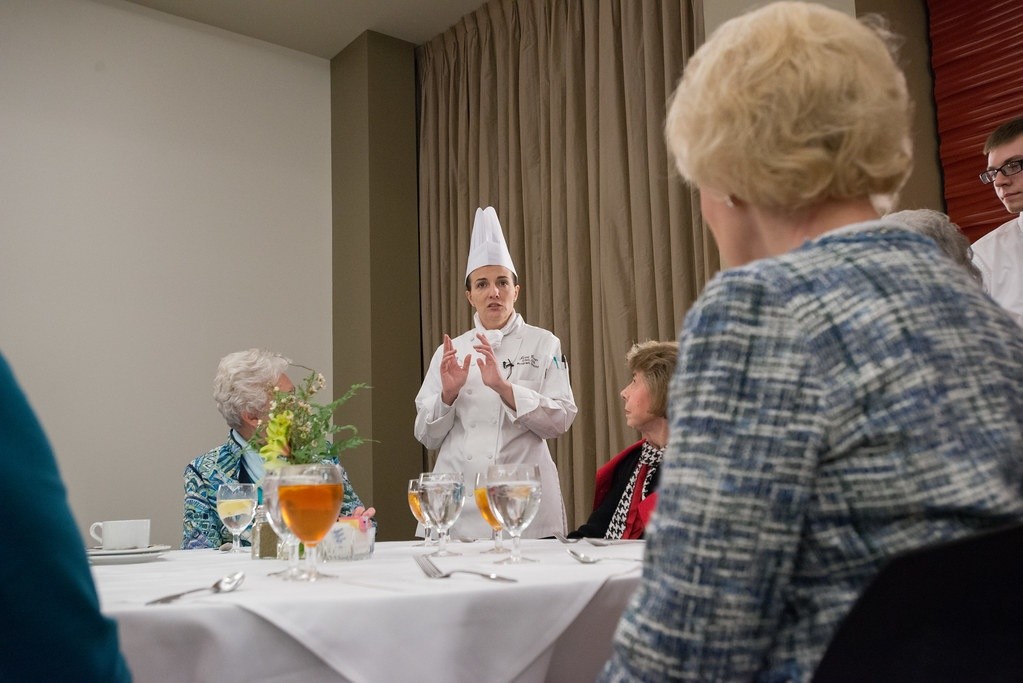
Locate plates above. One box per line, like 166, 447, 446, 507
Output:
87, 546, 172, 555
87, 552, 163, 566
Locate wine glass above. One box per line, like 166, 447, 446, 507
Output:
216, 483, 258, 555
407, 472, 466, 555
280, 464, 344, 580
487, 463, 543, 565
263, 475, 320, 578
475, 474, 512, 555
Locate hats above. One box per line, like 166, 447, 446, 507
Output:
465, 205, 518, 284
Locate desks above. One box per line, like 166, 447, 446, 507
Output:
87, 538, 646, 683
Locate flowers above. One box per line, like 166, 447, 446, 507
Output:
235, 370, 381, 463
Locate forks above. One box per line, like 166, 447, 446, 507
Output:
413, 555, 517, 583
553, 531, 584, 543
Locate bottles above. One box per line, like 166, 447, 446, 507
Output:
250, 505, 278, 560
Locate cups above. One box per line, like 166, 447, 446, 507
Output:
90, 519, 151, 549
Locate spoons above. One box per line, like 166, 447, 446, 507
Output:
146, 570, 245, 606
566, 548, 643, 564
584, 537, 644, 546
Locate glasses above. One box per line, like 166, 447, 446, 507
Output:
979, 159, 1022, 184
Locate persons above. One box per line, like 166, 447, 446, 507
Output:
594, 0, 1023, 683
181, 347, 378, 551
0, 352, 133, 683
413, 206, 579, 543
540, 339, 679, 541
966, 117, 1023, 327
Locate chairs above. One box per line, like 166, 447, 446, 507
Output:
809, 527, 1023, 683
